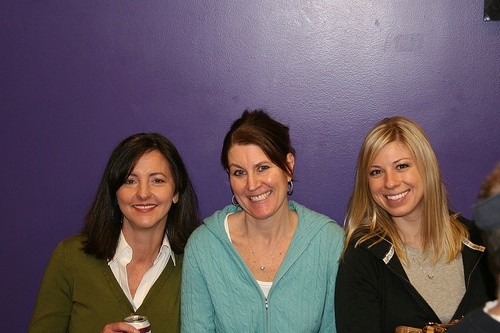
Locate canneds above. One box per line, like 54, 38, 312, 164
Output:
123, 315, 151, 333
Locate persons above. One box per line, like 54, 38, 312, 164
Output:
334, 116, 500, 333
27, 132, 200, 333
179, 110, 344, 333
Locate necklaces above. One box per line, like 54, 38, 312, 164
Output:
403, 237, 439, 279
247, 228, 277, 270
